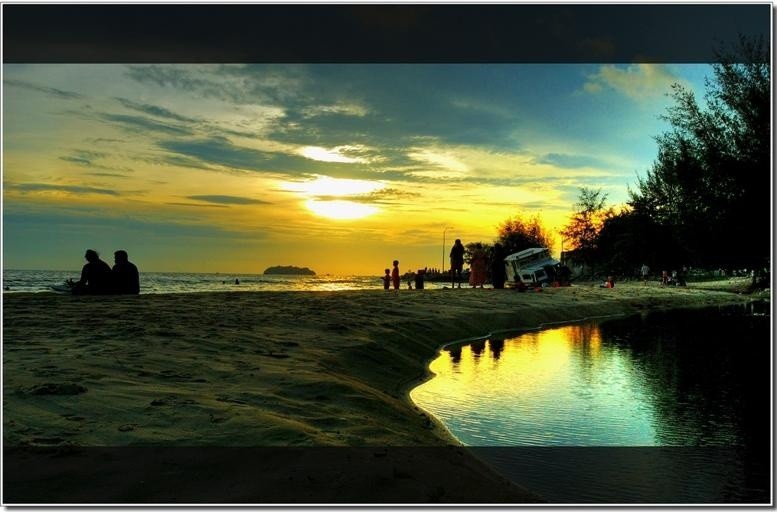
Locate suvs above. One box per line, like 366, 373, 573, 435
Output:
503, 247, 560, 287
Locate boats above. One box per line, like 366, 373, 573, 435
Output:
687, 273, 757, 294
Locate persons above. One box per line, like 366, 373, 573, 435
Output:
391, 261, 399, 289
662, 265, 688, 285
381, 269, 390, 289
408, 282, 412, 289
450, 239, 506, 289
71, 249, 115, 295
511, 275, 526, 292
113, 251, 139, 295
414, 270, 423, 289
640, 263, 652, 286
